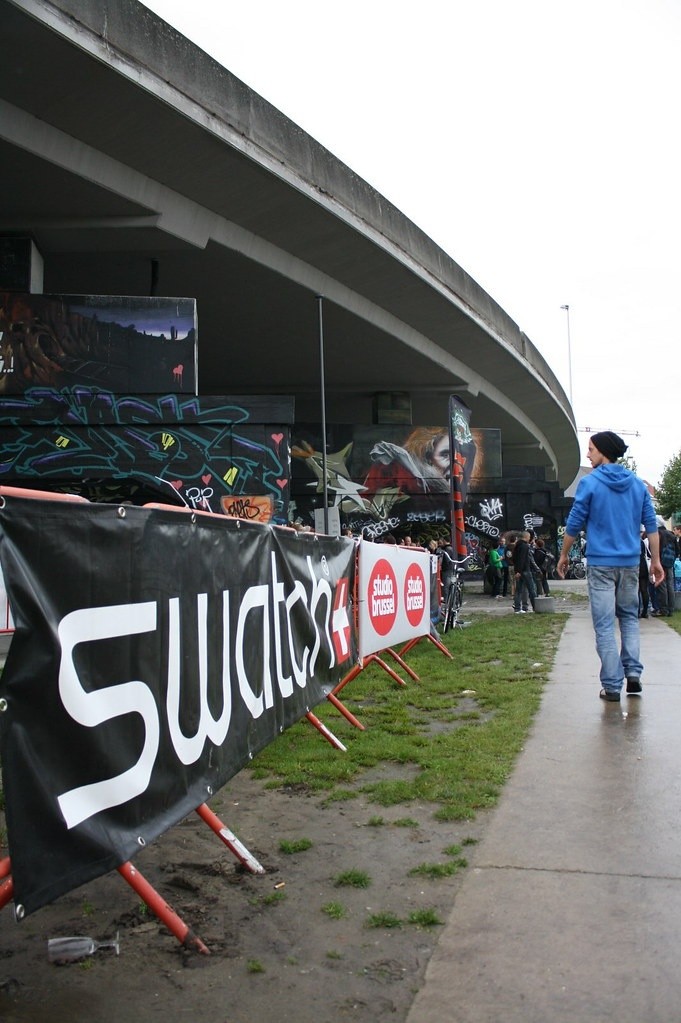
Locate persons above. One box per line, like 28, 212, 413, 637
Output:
342, 528, 353, 538
288, 521, 314, 532
557, 431, 665, 700
638, 515, 681, 618
384, 536, 455, 611
487, 530, 550, 613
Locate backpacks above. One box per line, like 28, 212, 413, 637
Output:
538, 550, 555, 573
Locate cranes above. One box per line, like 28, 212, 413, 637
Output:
577, 425, 642, 437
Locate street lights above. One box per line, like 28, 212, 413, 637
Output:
559, 302, 573, 405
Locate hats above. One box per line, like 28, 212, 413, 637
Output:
591, 432, 627, 462
656, 517, 666, 528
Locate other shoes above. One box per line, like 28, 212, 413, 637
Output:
641, 613, 648, 618
526, 608, 533, 612
495, 595, 503, 598
515, 610, 526, 614
668, 610, 673, 617
626, 681, 643, 692
600, 689, 620, 701
651, 610, 663, 616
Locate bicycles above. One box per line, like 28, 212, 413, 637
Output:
550, 556, 587, 581
436, 546, 479, 635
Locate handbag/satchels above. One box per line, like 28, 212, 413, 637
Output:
646, 558, 656, 584
661, 544, 676, 567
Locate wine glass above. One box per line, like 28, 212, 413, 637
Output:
48, 931, 122, 961
